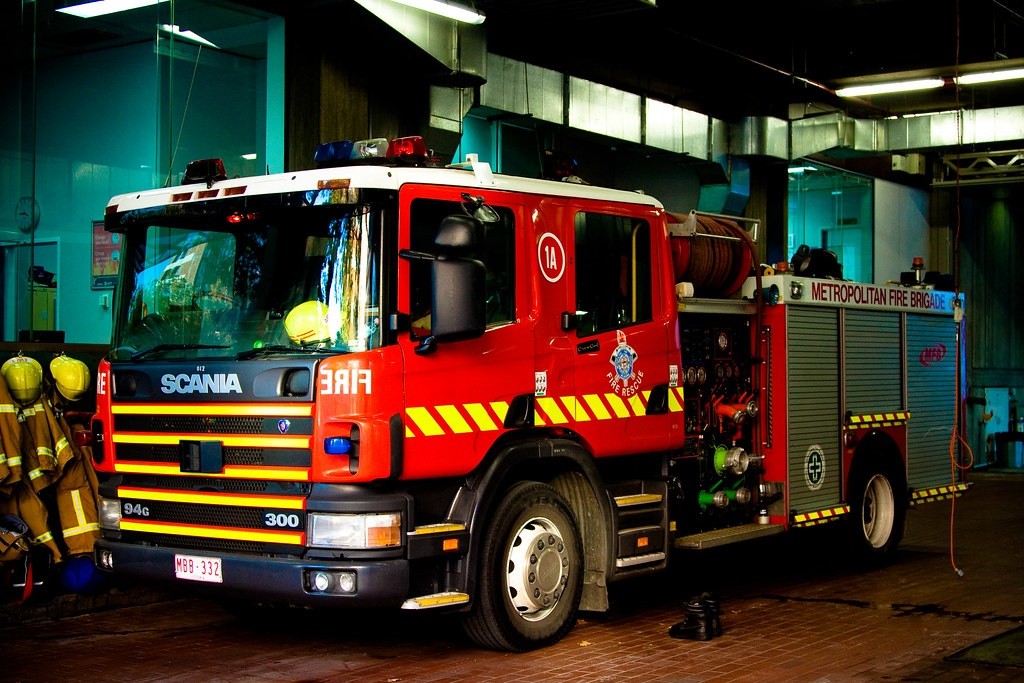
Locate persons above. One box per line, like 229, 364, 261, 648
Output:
412, 260, 507, 332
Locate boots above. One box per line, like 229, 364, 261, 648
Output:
704, 596, 723, 636
669, 601, 712, 641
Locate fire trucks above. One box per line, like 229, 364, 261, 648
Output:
89, 135, 971, 658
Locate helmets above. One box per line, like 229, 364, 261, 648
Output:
1, 357, 43, 405
50, 354, 90, 402
284, 301, 347, 344
